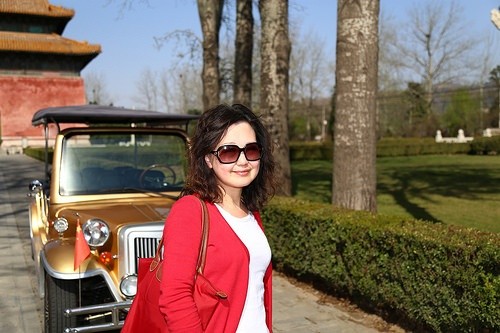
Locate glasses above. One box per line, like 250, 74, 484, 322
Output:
209, 142, 264, 164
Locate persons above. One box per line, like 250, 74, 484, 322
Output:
158, 104, 281, 333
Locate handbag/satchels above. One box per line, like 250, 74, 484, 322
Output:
117, 194, 231, 333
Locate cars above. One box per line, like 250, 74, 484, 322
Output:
26, 104, 207, 333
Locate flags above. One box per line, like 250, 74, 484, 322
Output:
75, 213, 91, 271
